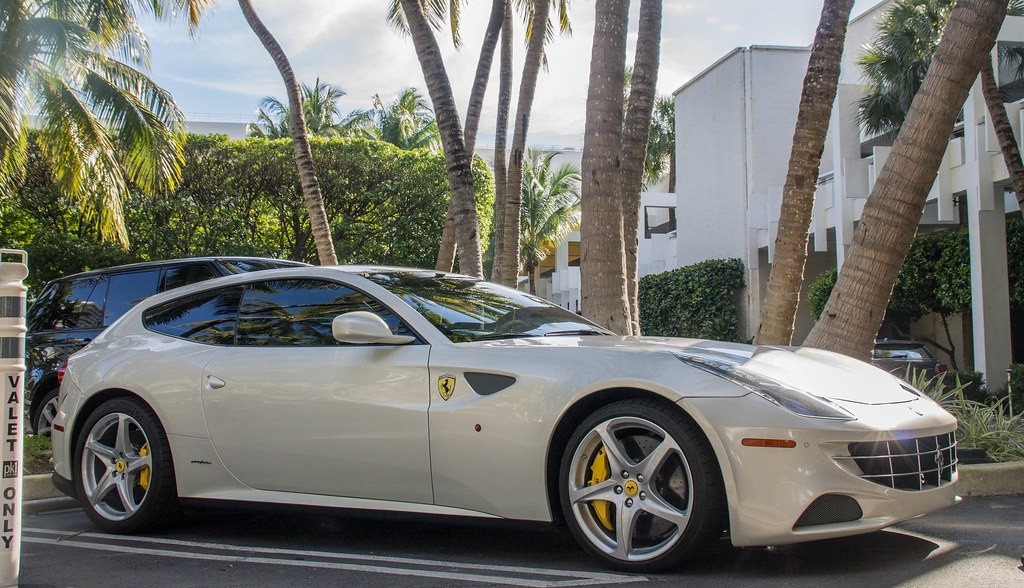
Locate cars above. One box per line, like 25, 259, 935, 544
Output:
49, 263, 964, 575
26, 256, 314, 440
873, 339, 952, 394
